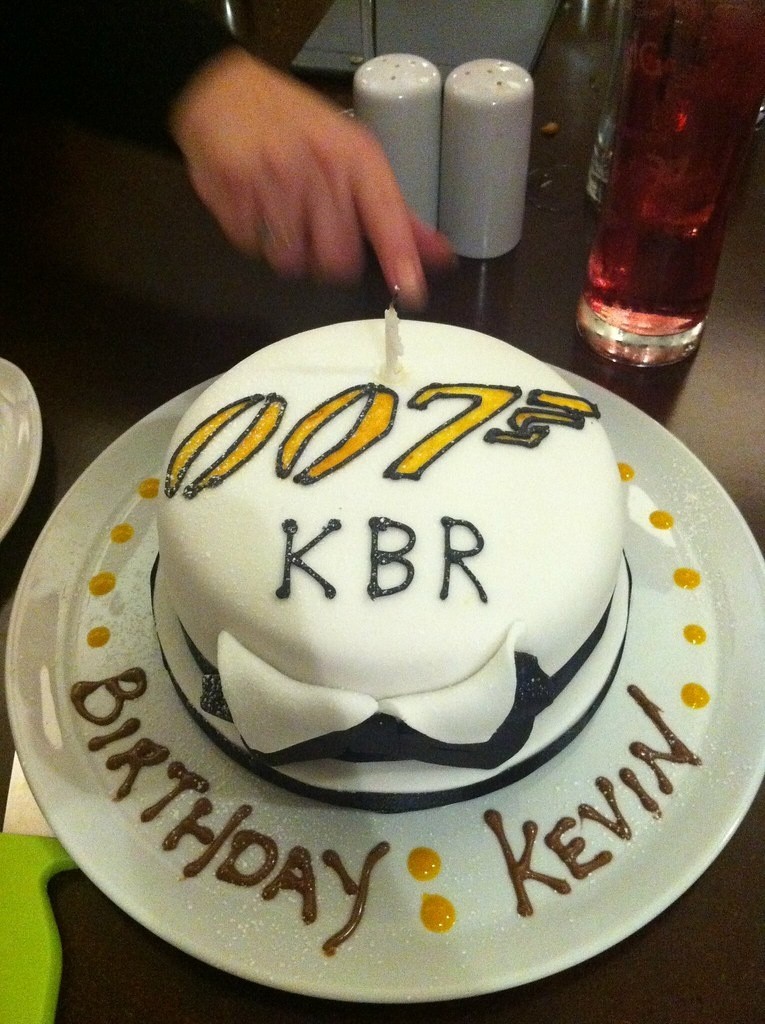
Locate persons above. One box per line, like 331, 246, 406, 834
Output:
2, 0, 457, 312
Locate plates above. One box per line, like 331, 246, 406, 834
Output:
0, 358, 42, 543
4, 359, 765, 1003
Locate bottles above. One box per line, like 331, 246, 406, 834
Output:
438, 57, 535, 260
353, 51, 442, 235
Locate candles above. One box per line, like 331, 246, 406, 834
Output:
384, 286, 407, 383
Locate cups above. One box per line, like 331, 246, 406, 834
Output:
585, 0, 631, 205
575, 0, 765, 368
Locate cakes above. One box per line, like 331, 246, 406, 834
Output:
149, 317, 634, 813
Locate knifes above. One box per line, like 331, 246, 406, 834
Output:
0, 749, 77, 1024
